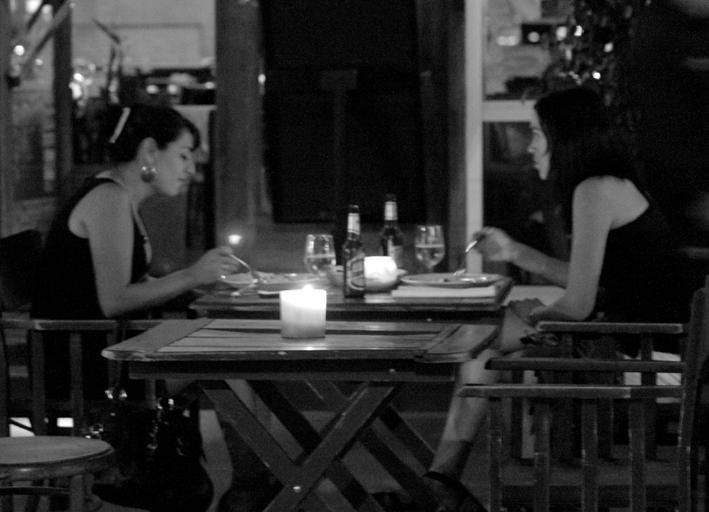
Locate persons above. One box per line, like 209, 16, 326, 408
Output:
368, 85, 694, 509
29, 96, 253, 511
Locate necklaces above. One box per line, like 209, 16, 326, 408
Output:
112, 166, 150, 247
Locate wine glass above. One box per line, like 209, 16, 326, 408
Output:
305, 234, 336, 296
414, 225, 446, 273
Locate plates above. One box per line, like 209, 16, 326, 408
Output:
220, 272, 319, 291
401, 271, 504, 288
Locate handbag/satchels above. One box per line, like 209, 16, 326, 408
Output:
90, 390, 213, 511
518, 318, 626, 463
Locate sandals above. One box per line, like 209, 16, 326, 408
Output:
373, 473, 464, 512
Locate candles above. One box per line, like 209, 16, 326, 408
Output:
280, 284, 326, 339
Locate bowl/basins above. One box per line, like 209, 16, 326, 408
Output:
365, 255, 408, 292
333, 265, 347, 287
396, 267, 407, 285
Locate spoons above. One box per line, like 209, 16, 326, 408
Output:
230, 253, 275, 280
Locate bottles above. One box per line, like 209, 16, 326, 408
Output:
379, 193, 406, 269
341, 204, 366, 298
